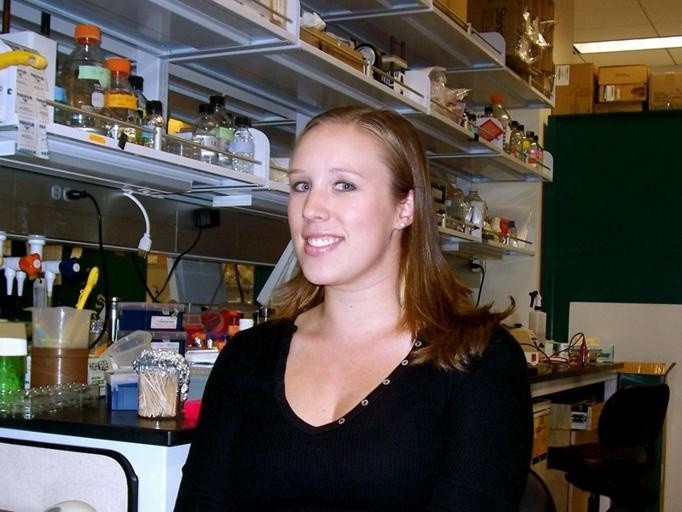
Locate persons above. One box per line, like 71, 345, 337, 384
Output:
174, 104, 533, 510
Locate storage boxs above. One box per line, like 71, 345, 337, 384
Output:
550, 61, 682, 115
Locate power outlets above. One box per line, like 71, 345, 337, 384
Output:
50, 185, 73, 204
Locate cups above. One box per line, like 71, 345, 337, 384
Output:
30, 305, 95, 388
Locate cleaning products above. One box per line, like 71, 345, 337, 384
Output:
526, 288, 548, 346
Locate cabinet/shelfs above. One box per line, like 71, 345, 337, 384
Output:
533, 393, 607, 511
1, 1, 559, 263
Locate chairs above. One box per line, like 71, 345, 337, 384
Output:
545, 381, 671, 512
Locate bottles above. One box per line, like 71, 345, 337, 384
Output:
463, 185, 519, 248
482, 94, 544, 171
57, 22, 257, 174
